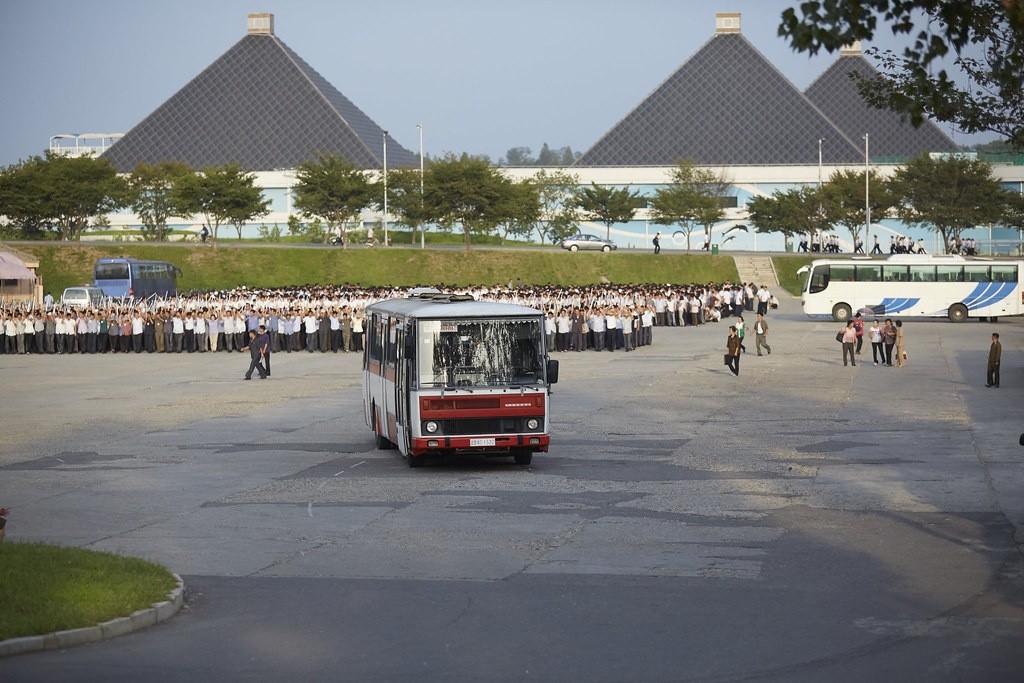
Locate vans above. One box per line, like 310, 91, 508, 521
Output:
61, 287, 104, 310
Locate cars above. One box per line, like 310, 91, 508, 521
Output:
561, 234, 617, 253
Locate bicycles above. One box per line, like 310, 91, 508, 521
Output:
330, 236, 351, 246
191, 233, 213, 246
361, 235, 380, 248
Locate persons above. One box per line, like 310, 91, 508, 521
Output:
367, 226, 378, 247
201, 223, 209, 242
387, 234, 392, 247
985, 333, 1002, 388
868, 318, 905, 368
839, 312, 864, 366
702, 231, 710, 252
334, 222, 343, 246
855, 237, 864, 254
0, 281, 779, 357
948, 235, 978, 256
871, 234, 883, 254
723, 326, 741, 378
797, 233, 842, 253
587, 236, 591, 241
241, 325, 270, 380
652, 233, 662, 254
890, 235, 927, 254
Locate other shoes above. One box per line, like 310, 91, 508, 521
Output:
994, 384, 1000, 388
894, 365, 898, 367
768, 351, 770, 354
856, 351, 860, 354
758, 354, 762, 356
743, 347, 745, 352
259, 376, 266, 379
257, 374, 260, 375
985, 383, 995, 387
731, 372, 737, 376
852, 364, 855, 366
888, 364, 892, 366
899, 365, 902, 367
844, 363, 847, 366
244, 377, 251, 380
873, 363, 877, 365
266, 373, 270, 376
883, 363, 885, 366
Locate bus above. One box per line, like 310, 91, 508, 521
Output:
362, 287, 559, 467
796, 253, 1024, 322
93, 257, 184, 299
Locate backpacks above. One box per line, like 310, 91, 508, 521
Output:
853, 323, 860, 332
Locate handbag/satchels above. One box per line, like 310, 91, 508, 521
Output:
880, 332, 885, 342
724, 354, 729, 365
896, 352, 906, 360
653, 239, 655, 244
836, 332, 844, 343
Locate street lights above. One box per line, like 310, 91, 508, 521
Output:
862, 133, 869, 258
817, 137, 827, 252
416, 123, 425, 248
383, 131, 388, 247
